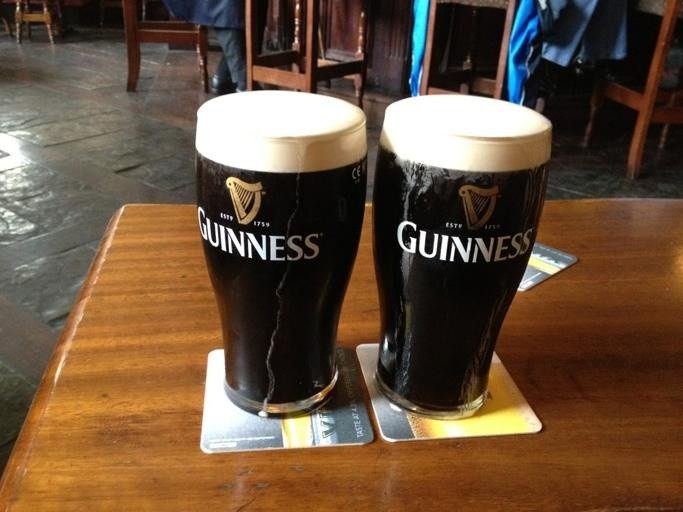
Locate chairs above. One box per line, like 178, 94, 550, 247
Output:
0, 2, 151, 44
240, 1, 680, 178
123, 0, 298, 93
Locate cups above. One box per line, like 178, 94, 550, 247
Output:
193, 90, 368, 416
372, 93, 554, 417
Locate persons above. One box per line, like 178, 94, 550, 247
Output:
160, 1, 270, 93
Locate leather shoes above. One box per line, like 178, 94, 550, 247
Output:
211, 77, 234, 94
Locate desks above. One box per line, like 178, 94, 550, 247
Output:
0, 198, 683, 511
